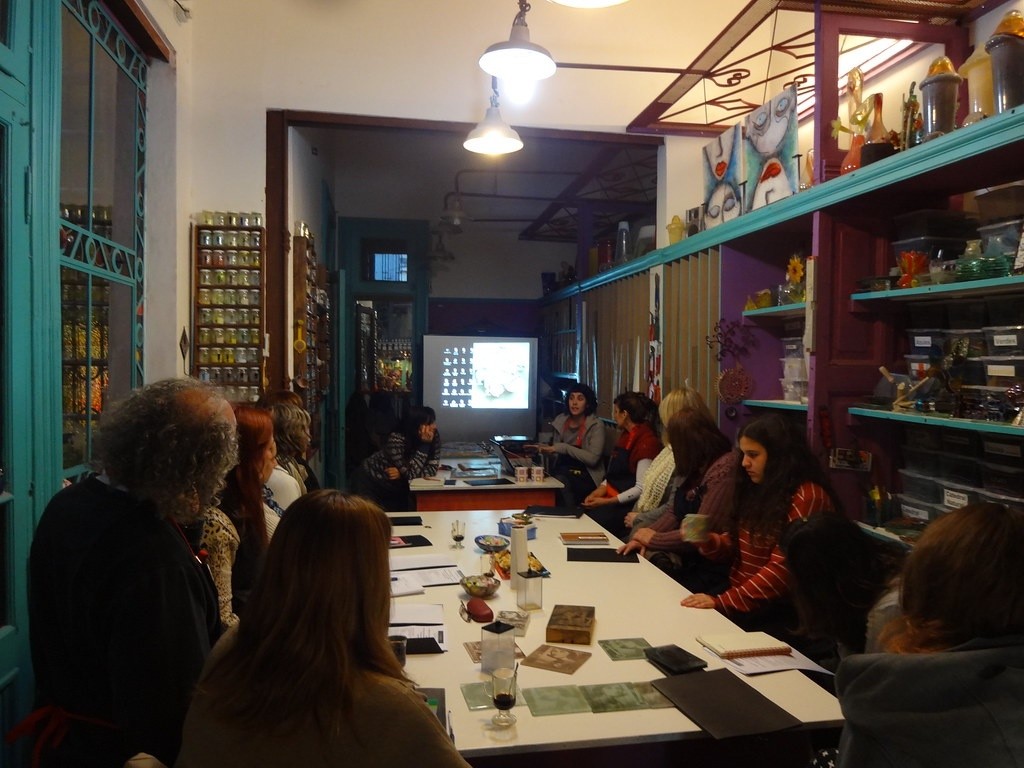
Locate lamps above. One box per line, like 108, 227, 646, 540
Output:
438, 169, 582, 225
424, 218, 536, 264
460, 58, 687, 157
429, 192, 558, 235
477, 0, 558, 85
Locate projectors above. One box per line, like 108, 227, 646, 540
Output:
494, 435, 533, 452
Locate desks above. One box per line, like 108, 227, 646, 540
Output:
408, 456, 566, 511
381, 509, 845, 768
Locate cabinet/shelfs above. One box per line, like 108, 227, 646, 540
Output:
59, 218, 112, 306
715, 102, 1024, 555
318, 264, 332, 388
544, 283, 582, 419
293, 235, 322, 462
189, 222, 269, 405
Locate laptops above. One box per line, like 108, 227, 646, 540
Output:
490, 439, 550, 478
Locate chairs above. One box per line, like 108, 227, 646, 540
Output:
835, 646, 1024, 768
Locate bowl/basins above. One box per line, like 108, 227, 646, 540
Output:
460, 575, 501, 597
475, 535, 510, 553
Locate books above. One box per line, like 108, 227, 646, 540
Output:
389, 516, 422, 526
560, 532, 610, 545
405, 637, 444, 655
695, 632, 792, 659
390, 535, 432, 549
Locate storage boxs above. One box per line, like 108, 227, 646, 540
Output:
979, 431, 1024, 468
958, 385, 1009, 401
905, 424, 942, 450
976, 219, 1024, 257
539, 415, 555, 432
892, 208, 980, 241
981, 325, 1024, 356
897, 468, 943, 504
685, 204, 707, 223
890, 235, 971, 266
898, 443, 936, 473
981, 356, 1024, 387
978, 488, 1024, 506
978, 460, 1024, 500
903, 328, 947, 354
538, 432, 555, 445
517, 570, 542, 611
497, 522, 537, 540
942, 329, 988, 358
934, 504, 955, 518
481, 621, 515, 673
934, 478, 979, 510
936, 450, 984, 488
941, 428, 984, 458
964, 356, 987, 386
545, 604, 596, 645
896, 493, 935, 522
962, 180, 1024, 222
685, 220, 705, 237
903, 354, 931, 380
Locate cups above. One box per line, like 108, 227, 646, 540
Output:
531, 466, 544, 485
515, 467, 528, 484
388, 635, 407, 667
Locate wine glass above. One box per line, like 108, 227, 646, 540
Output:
492, 668, 517, 727
451, 520, 466, 550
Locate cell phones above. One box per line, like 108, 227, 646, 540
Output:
444, 479, 457, 486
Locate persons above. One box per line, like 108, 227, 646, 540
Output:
255, 391, 321, 537
778, 514, 910, 694
28, 378, 240, 768
176, 489, 470, 768
548, 649, 577, 667
351, 406, 441, 509
594, 684, 642, 708
618, 639, 649, 657
200, 408, 278, 628
834, 501, 1024, 768
577, 387, 710, 542
680, 416, 840, 648
521, 384, 605, 506
615, 409, 741, 592
